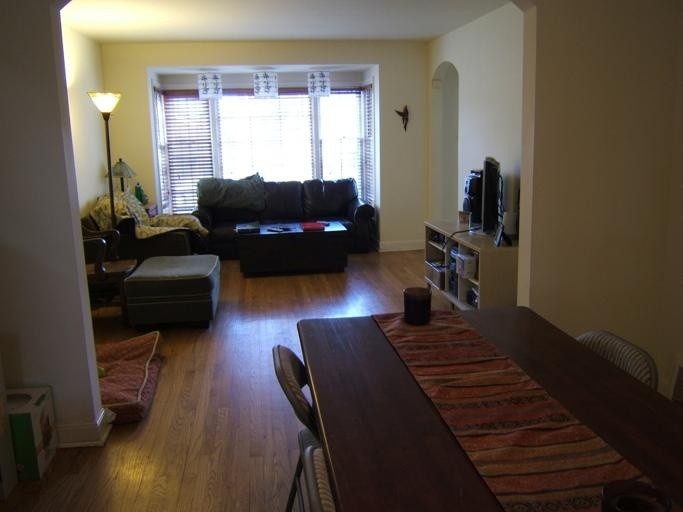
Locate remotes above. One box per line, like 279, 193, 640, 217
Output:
267, 228, 283, 231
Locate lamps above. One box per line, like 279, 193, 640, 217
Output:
86, 90, 126, 232
103, 157, 138, 193
196, 71, 330, 101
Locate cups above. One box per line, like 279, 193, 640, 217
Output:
501, 210, 517, 236
403, 287, 433, 326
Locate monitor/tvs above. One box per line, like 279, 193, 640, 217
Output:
481, 156, 504, 234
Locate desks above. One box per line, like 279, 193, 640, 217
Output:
294, 309, 682, 512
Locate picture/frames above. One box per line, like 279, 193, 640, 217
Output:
493, 223, 504, 247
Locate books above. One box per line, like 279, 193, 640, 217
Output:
301, 222, 324, 230
316, 220, 330, 226
238, 220, 261, 232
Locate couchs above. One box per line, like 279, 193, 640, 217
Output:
193, 172, 378, 261
81, 191, 209, 256
121, 252, 222, 333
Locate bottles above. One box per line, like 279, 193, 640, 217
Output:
134, 182, 142, 203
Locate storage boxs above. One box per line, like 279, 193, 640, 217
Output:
5, 385, 58, 482
423, 257, 454, 291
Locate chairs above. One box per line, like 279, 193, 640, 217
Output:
270, 343, 323, 512
300, 440, 335, 512
80, 224, 139, 329
575, 328, 657, 396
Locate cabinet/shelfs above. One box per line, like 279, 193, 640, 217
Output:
422, 218, 518, 310
142, 202, 157, 218
